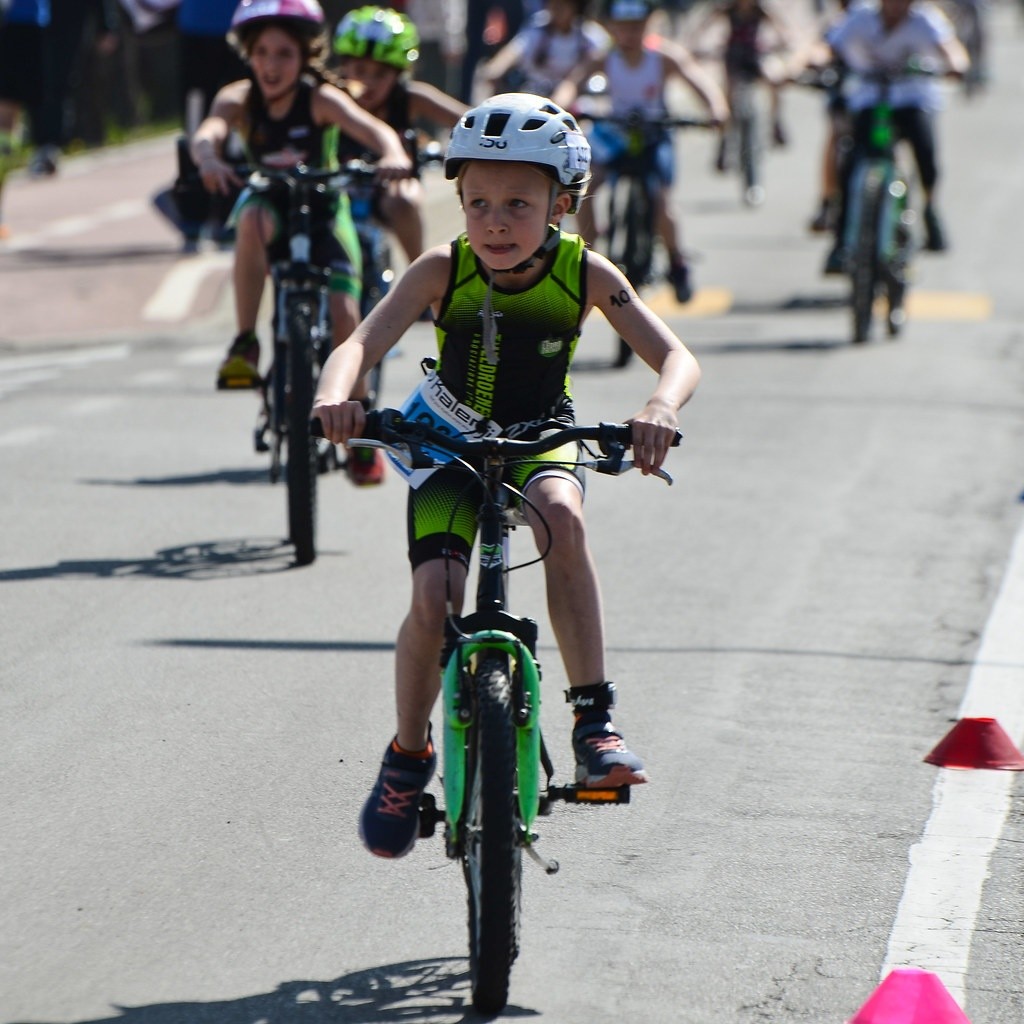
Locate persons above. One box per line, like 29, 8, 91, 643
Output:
0, 0, 994, 323
186, 0, 426, 485
304, 89, 704, 854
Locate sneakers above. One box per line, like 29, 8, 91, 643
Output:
357, 719, 437, 858
346, 445, 387, 487
572, 712, 649, 788
212, 326, 261, 384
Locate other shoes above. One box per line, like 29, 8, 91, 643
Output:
808, 199, 842, 231
921, 208, 944, 251
666, 261, 694, 304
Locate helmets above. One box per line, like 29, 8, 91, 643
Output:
445, 92, 591, 275
334, 5, 420, 112
232, 1, 324, 100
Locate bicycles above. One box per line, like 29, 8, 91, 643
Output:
332, 149, 445, 412
302, 406, 684, 1016
175, 128, 424, 566
481, 71, 613, 107
686, 44, 798, 209
567, 110, 721, 366
801, 60, 981, 344
